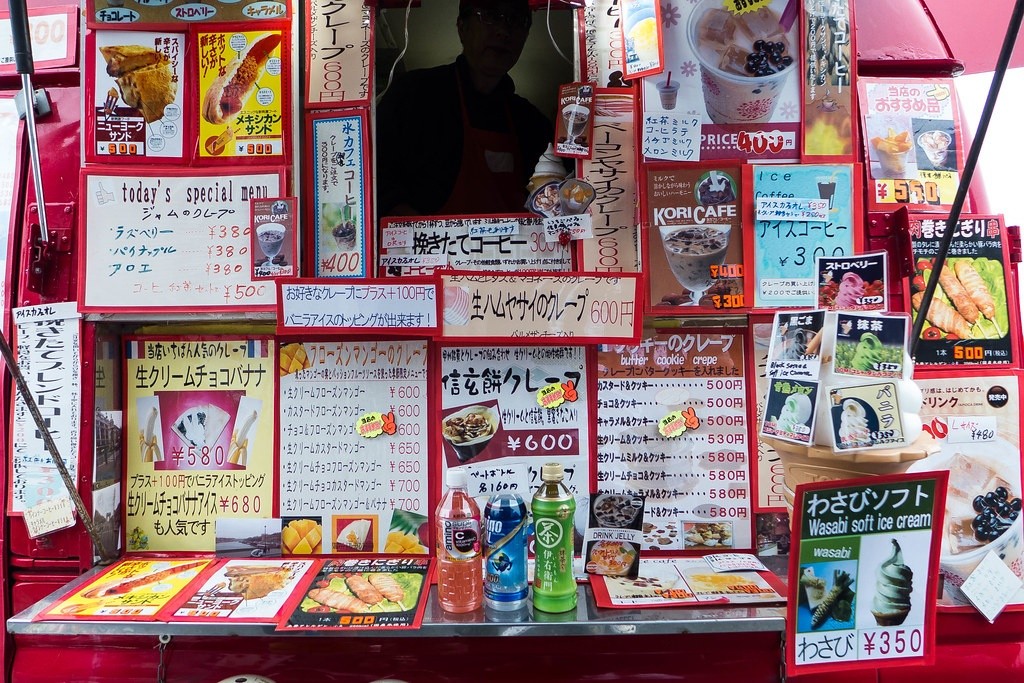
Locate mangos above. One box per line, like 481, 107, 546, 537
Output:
384, 532, 426, 555
281, 519, 322, 555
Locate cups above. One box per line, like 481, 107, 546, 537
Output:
622, 8, 659, 64
815, 176, 837, 210
656, 80, 681, 110
687, 0, 799, 134
874, 149, 912, 175
530, 178, 596, 219
917, 130, 952, 169
906, 450, 1024, 605
331, 221, 356, 252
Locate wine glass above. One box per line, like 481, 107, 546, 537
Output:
659, 224, 731, 308
256, 223, 286, 270
562, 103, 590, 145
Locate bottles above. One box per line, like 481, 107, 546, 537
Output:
531, 463, 578, 614
436, 467, 484, 614
484, 494, 531, 609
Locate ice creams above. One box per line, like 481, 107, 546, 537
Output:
869, 538, 913, 627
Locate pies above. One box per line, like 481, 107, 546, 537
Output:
204, 33, 279, 127
